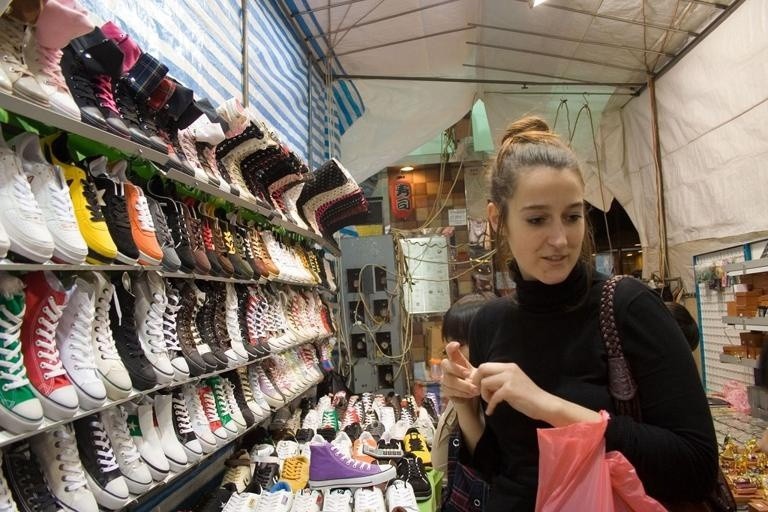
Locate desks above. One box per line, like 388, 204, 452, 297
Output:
418, 464, 445, 512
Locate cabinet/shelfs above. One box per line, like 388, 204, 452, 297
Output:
719, 257, 768, 370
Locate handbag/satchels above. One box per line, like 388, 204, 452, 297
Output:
442, 461, 500, 512
661, 465, 738, 512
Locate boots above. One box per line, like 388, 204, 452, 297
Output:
1, 0, 247, 153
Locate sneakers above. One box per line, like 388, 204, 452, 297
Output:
355, 392, 438, 512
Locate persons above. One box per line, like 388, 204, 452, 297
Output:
440, 115, 717, 512
440, 294, 499, 364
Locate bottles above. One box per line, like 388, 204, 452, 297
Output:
743, 242, 752, 261
758, 306, 767, 317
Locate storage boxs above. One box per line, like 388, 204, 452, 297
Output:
424, 327, 445, 368
